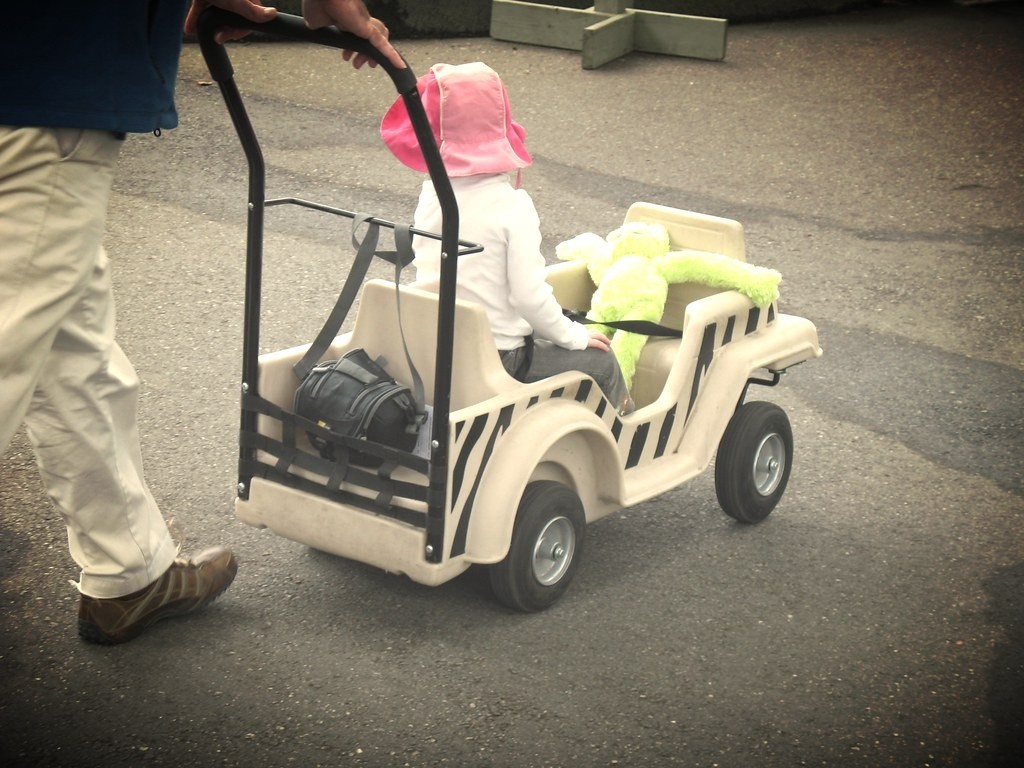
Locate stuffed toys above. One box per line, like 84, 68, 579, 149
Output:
557, 223, 781, 391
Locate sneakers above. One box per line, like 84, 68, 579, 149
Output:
78, 543, 237, 643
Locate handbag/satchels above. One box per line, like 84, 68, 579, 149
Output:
295, 345, 428, 469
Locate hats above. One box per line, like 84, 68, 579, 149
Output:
380, 61, 532, 189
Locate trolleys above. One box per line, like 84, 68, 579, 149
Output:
198, 7, 824, 613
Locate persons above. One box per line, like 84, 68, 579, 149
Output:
0, 1, 406, 644
380, 62, 674, 468
